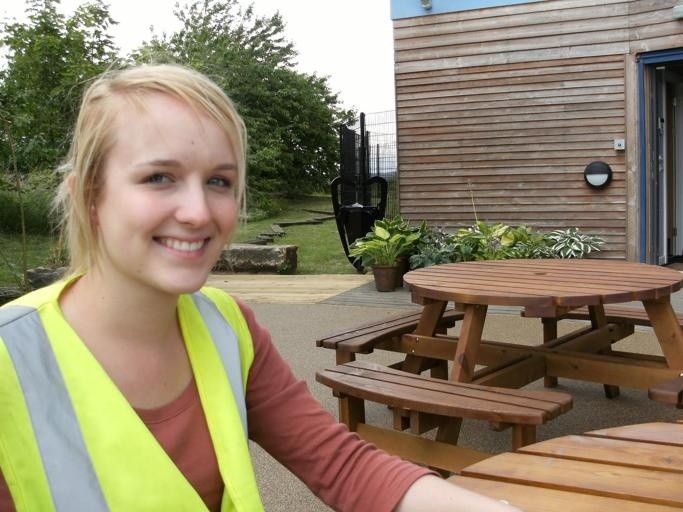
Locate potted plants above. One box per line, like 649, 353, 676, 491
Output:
348, 214, 426, 293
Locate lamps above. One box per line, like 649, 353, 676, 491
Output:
585, 161, 612, 188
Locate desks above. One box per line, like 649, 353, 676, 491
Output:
447, 420, 683, 512
403, 259, 683, 478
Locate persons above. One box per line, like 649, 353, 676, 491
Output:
0, 62, 520, 512
